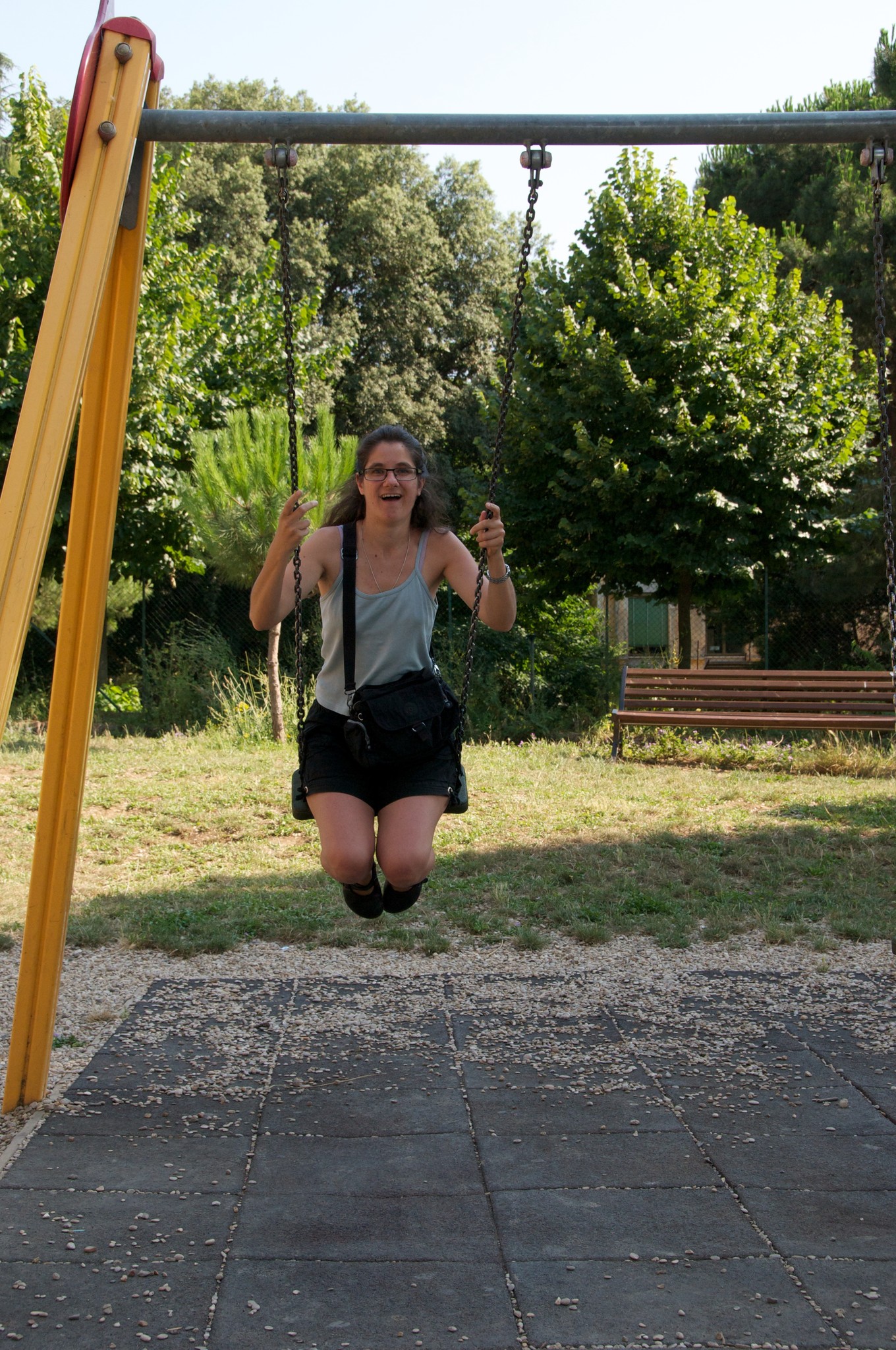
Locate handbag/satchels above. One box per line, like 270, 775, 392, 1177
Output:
348, 668, 467, 769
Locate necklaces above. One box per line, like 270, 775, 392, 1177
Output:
361, 524, 409, 593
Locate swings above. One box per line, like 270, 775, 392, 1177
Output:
264, 139, 553, 823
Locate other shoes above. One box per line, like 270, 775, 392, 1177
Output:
382, 877, 429, 914
341, 860, 384, 918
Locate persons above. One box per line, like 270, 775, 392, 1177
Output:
249, 424, 517, 918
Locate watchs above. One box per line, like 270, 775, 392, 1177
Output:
487, 564, 511, 584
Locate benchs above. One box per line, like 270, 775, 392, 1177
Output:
704, 659, 753, 669
611, 665, 896, 764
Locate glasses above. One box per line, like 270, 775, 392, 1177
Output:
359, 467, 422, 482
